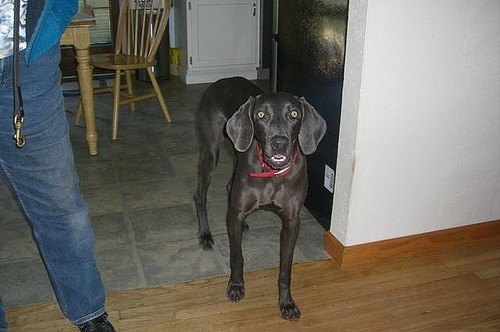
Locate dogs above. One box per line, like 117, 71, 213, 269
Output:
192, 75, 327, 322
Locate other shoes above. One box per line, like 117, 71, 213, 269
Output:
77, 312, 117, 332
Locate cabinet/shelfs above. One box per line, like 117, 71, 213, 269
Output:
175, 0, 260, 85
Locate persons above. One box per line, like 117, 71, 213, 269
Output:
0, 0, 115, 332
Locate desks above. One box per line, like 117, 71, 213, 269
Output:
60, 10, 97, 155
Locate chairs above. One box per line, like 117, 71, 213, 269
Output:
75, 0, 171, 140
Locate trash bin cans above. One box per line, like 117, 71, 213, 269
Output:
129, 8, 172, 83
